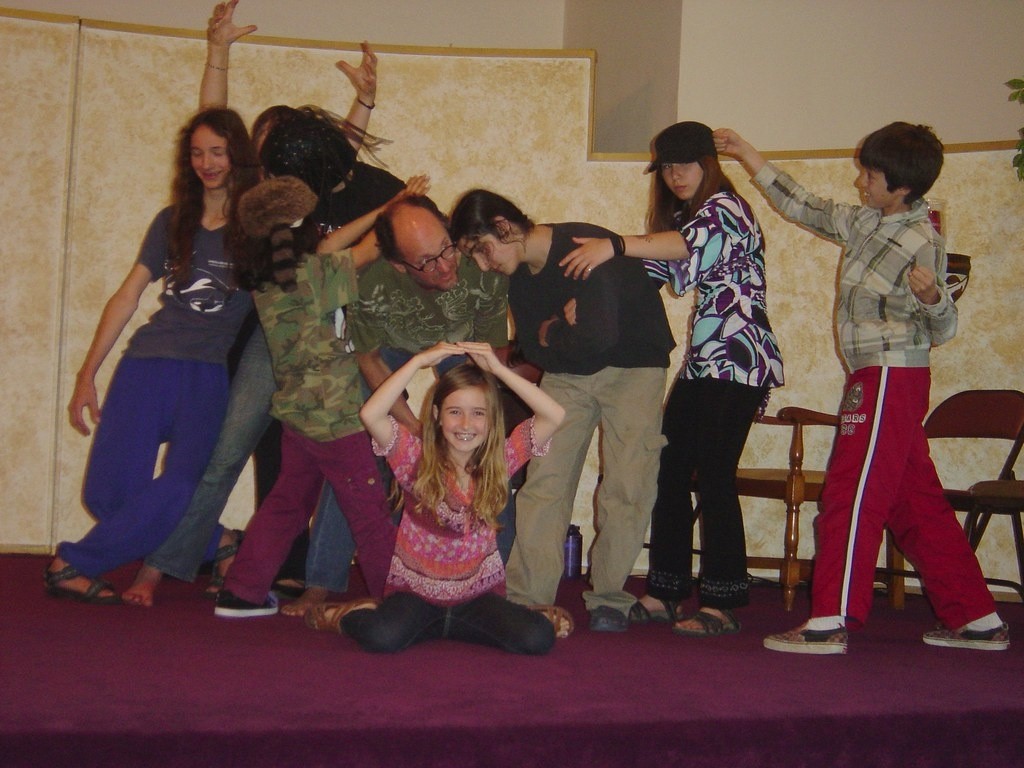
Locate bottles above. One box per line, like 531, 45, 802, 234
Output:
561, 524, 583, 579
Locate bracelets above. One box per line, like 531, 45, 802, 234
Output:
609, 235, 626, 258
357, 96, 376, 109
206, 62, 230, 71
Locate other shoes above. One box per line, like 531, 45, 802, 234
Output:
763, 611, 848, 654
591, 602, 623, 632
280, 586, 326, 618
923, 612, 1010, 652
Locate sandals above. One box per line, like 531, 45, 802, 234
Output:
675, 607, 742, 635
209, 527, 244, 596
630, 594, 684, 623
43, 562, 115, 605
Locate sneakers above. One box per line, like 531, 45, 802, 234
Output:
213, 586, 280, 616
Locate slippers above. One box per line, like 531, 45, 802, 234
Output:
528, 606, 575, 640
307, 596, 378, 632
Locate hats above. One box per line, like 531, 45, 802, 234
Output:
648, 122, 717, 172
239, 177, 316, 290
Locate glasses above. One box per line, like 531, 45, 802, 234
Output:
393, 242, 459, 274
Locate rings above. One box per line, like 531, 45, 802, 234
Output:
585, 266, 592, 273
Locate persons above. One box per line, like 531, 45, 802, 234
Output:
308, 340, 574, 651
558, 120, 784, 635
448, 188, 678, 633
43, 1, 546, 616
713, 121, 1013, 653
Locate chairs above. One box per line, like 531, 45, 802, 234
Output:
642, 389, 1024, 610
